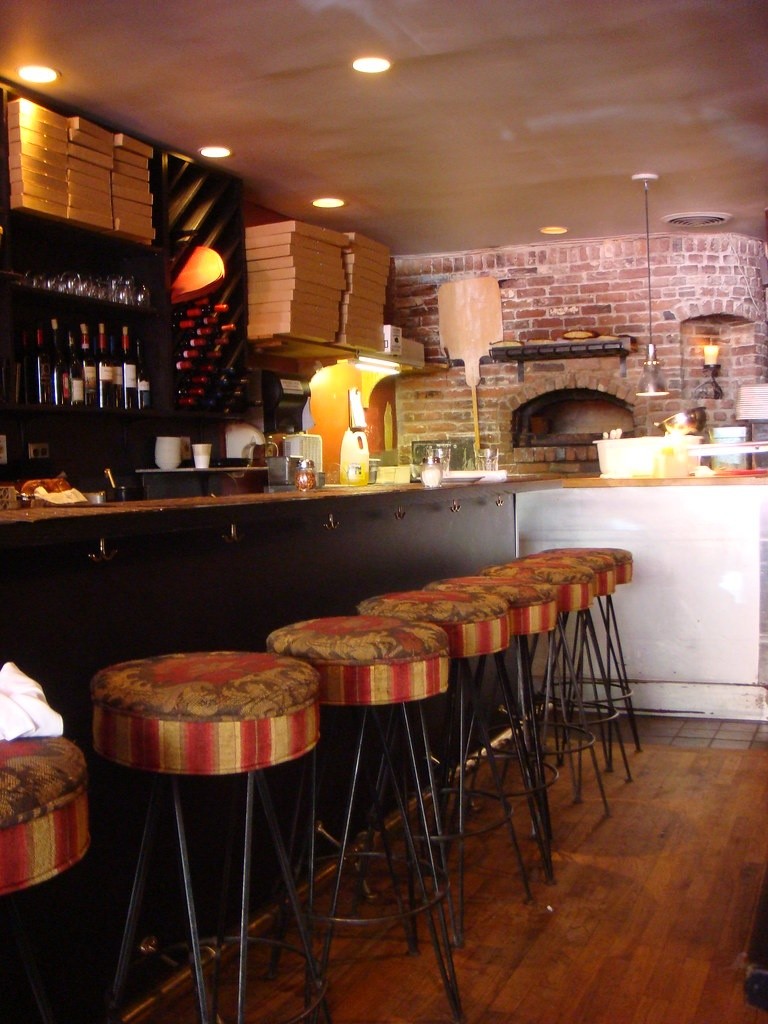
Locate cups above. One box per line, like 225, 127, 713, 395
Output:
426, 446, 452, 475
24, 267, 150, 307
192, 444, 212, 469
477, 449, 499, 472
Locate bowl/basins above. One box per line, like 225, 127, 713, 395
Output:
657, 407, 706, 436
154, 437, 182, 470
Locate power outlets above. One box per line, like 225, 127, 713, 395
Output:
28, 442, 51, 460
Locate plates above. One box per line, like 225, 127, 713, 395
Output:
441, 476, 485, 483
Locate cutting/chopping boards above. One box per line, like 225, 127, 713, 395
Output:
437, 278, 503, 386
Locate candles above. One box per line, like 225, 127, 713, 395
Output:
702, 344, 719, 364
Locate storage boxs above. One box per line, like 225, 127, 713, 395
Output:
243, 219, 392, 353
7, 98, 158, 248
383, 324, 403, 355
360, 339, 425, 366
593, 434, 704, 477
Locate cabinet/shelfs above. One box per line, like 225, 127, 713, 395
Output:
0, 74, 253, 423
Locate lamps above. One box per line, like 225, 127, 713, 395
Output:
631, 174, 670, 397
346, 355, 403, 377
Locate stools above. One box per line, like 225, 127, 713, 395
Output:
95, 548, 643, 1024
0, 735, 89, 1024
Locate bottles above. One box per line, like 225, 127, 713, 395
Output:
420, 456, 441, 488
340, 427, 369, 486
295, 458, 316, 492
1, 311, 155, 411
172, 297, 264, 416
